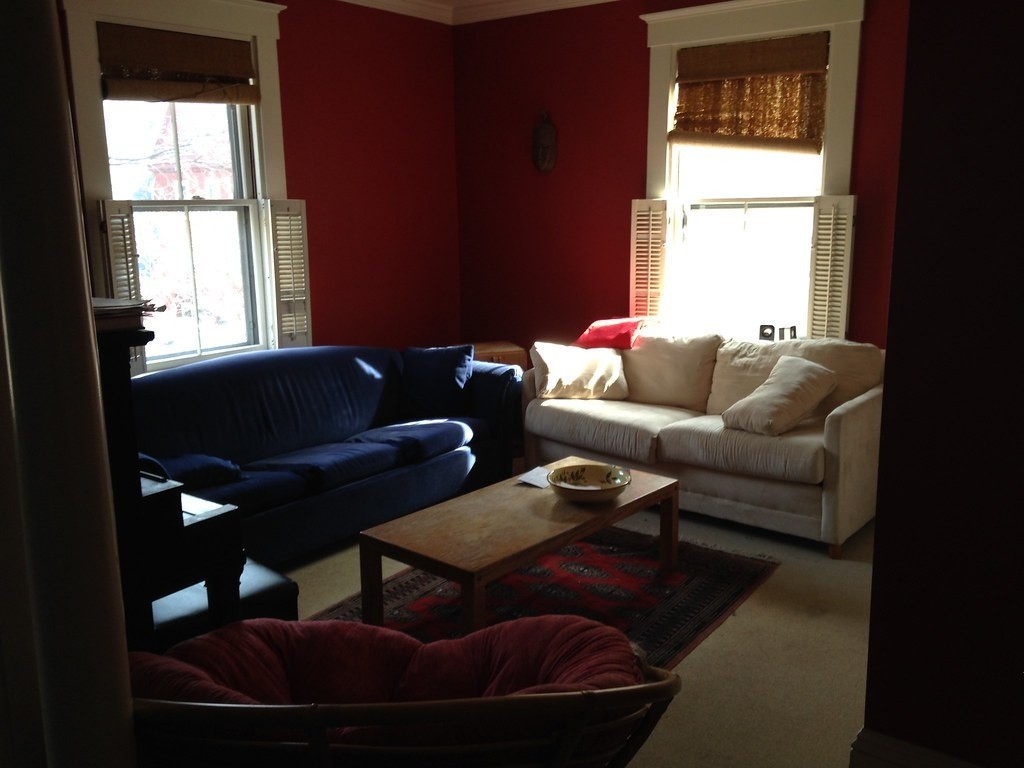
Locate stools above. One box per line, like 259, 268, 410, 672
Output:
153, 558, 301, 647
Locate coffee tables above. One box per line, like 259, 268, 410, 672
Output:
359, 456, 680, 635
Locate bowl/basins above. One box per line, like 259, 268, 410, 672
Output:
547, 464, 632, 505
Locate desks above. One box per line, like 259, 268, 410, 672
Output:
471, 342, 528, 375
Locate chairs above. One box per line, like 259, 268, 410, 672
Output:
130, 615, 683, 768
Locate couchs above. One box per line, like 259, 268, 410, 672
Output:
519, 327, 886, 558
131, 345, 523, 570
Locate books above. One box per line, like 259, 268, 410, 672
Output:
95, 311, 145, 335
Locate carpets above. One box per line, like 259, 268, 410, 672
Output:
305, 526, 780, 670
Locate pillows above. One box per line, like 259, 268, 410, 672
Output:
163, 451, 244, 490
398, 343, 475, 416
529, 341, 630, 400
722, 354, 839, 434
572, 317, 646, 349
